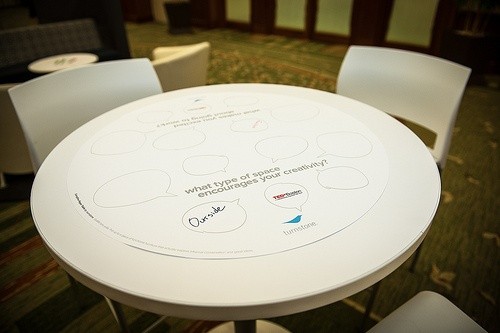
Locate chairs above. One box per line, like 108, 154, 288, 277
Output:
7, 58, 166, 299
366, 291, 489, 333
334, 43, 472, 269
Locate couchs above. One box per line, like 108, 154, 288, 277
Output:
0, 40, 211, 188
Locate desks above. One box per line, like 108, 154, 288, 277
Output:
28, 80, 445, 333
28, 52, 98, 72
